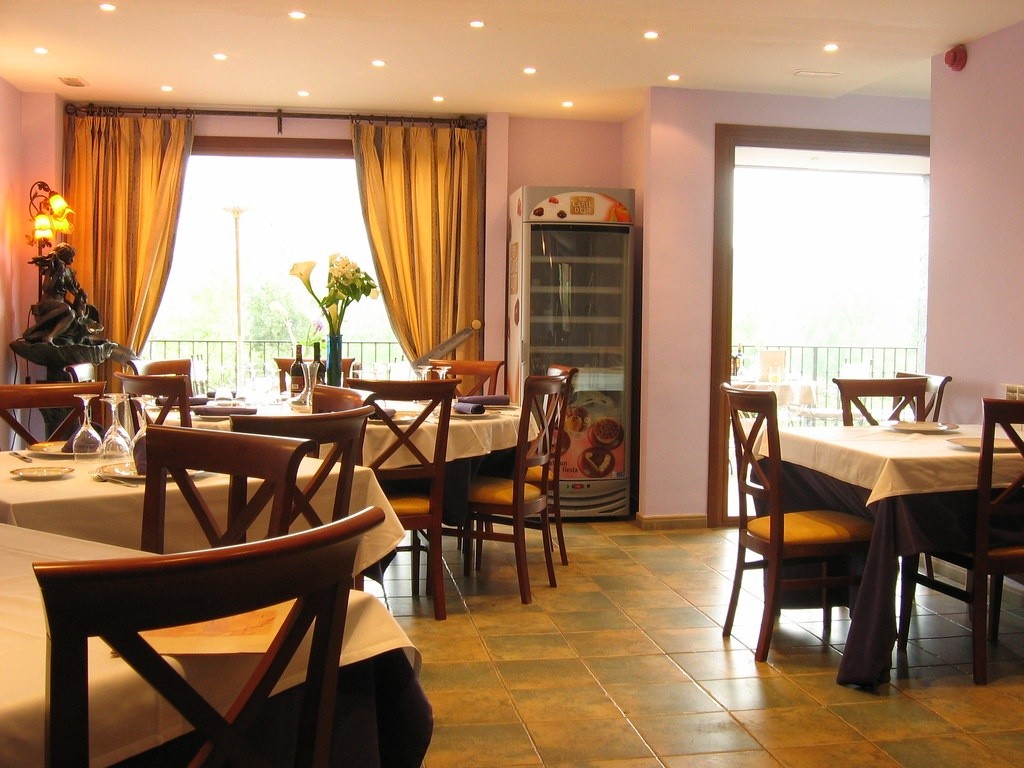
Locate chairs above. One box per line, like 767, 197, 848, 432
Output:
0, 357, 579, 621
720, 382, 876, 660
898, 398, 1024, 685
832, 372, 952, 579
32, 505, 385, 768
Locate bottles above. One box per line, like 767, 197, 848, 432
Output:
289, 345, 305, 397
310, 342, 325, 390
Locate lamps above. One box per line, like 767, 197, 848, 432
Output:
25, 180, 74, 301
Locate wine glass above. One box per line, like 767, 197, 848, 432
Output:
413, 365, 455, 408
72, 393, 157, 465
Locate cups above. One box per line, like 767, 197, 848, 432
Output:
593, 418, 623, 443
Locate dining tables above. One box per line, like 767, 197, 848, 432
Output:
739, 422, 1024, 686
0, 402, 540, 767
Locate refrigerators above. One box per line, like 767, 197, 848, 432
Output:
508, 186, 636, 518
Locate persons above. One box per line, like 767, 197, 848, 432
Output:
24, 242, 88, 346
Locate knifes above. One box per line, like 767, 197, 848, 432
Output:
9, 452, 33, 463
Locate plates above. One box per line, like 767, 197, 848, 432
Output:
27, 441, 99, 454
96, 462, 204, 477
889, 422, 958, 431
577, 447, 614, 478
435, 408, 492, 416
588, 424, 624, 449
10, 467, 74, 480
946, 437, 1018, 448
536, 427, 570, 457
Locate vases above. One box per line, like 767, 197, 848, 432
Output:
328, 335, 341, 386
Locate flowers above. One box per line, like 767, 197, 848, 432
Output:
290, 255, 381, 335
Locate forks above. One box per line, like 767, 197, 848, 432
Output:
88, 468, 138, 486
